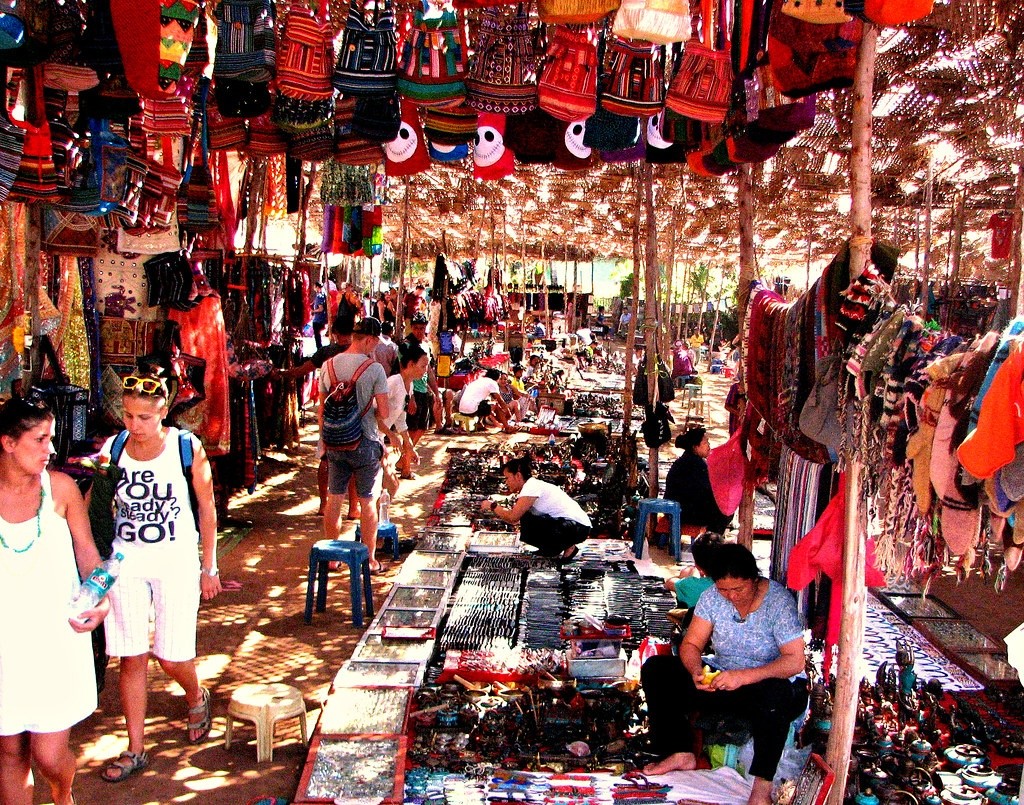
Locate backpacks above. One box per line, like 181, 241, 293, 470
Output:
322, 357, 375, 452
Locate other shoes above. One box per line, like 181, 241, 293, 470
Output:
533, 549, 555, 558
425, 417, 534, 434
559, 545, 579, 564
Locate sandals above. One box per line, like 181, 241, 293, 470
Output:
188, 685, 212, 745
101, 747, 150, 782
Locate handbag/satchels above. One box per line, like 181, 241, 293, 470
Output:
24, 334, 89, 468
1, 0, 870, 257
641, 402, 671, 449
632, 328, 674, 405
707, 425, 745, 517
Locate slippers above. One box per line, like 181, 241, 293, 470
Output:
329, 560, 343, 571
395, 464, 403, 470
361, 561, 389, 574
400, 471, 415, 480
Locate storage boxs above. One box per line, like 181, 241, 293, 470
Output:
572, 637, 624, 659
538, 388, 548, 397
510, 334, 528, 348
565, 648, 628, 678
537, 398, 565, 414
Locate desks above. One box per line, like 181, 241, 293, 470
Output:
437, 354, 510, 389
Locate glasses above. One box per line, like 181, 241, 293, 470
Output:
404, 394, 410, 412
122, 376, 168, 406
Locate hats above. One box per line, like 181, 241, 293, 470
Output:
353, 317, 381, 336
412, 312, 427, 323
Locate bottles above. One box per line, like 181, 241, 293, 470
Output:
379, 489, 390, 526
580, 647, 616, 656
63, 552, 124, 624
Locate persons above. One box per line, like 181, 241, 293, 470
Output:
526, 318, 545, 340
308, 281, 441, 352
458, 368, 529, 432
618, 308, 632, 332
512, 354, 543, 411
710, 335, 740, 379
549, 309, 581, 338
594, 306, 612, 340
636, 544, 807, 805
91, 364, 225, 782
691, 326, 704, 365
664, 428, 734, 544
479, 451, 595, 562
664, 531, 723, 609
0, 397, 114, 805
274, 314, 461, 522
722, 370, 746, 439
549, 331, 603, 367
316, 316, 393, 573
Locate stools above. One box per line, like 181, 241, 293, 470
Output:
449, 412, 478, 435
724, 368, 732, 378
223, 683, 309, 763
687, 397, 713, 427
354, 522, 399, 558
655, 517, 707, 552
724, 721, 795, 770
686, 415, 705, 431
683, 423, 705, 434
683, 383, 702, 406
711, 365, 724, 374
631, 498, 682, 561
304, 539, 375, 630
678, 375, 692, 387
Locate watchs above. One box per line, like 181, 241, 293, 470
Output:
490, 501, 498, 512
